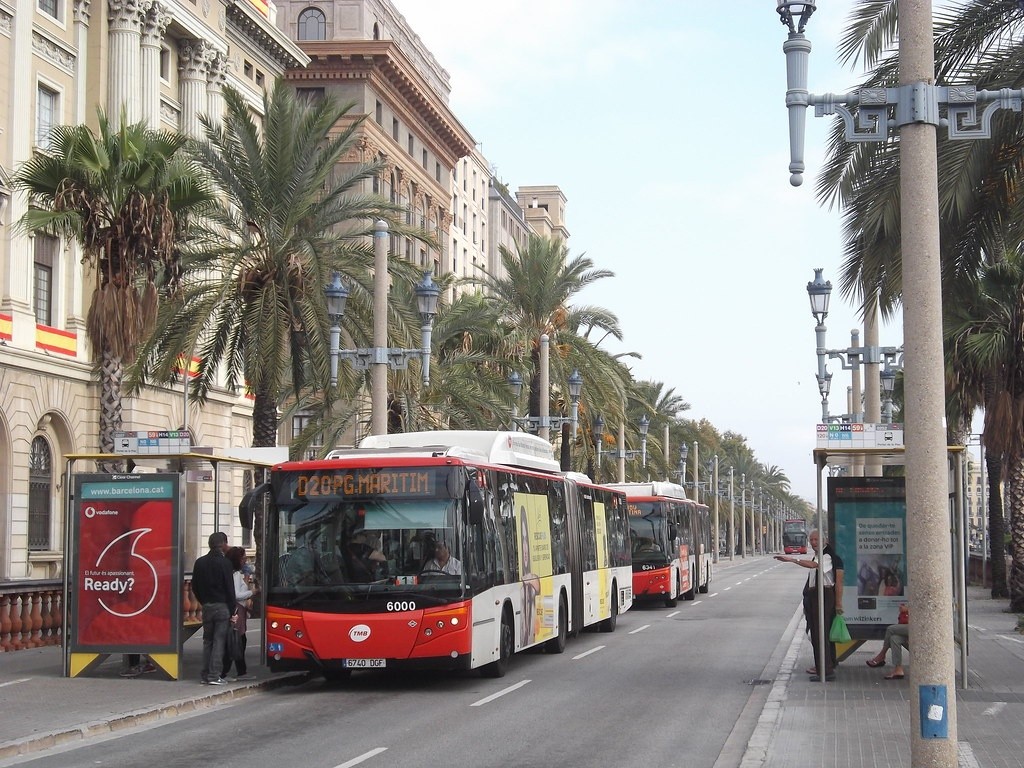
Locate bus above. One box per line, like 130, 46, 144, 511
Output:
601, 481, 713, 608
783, 519, 808, 554
238, 430, 633, 681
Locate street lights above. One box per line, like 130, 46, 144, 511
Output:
509, 333, 583, 443
777, 0, 959, 768
805, 267, 883, 478
702, 454, 732, 563
663, 423, 689, 486
323, 219, 439, 436
685, 441, 712, 505
590, 402, 649, 484
718, 466, 799, 561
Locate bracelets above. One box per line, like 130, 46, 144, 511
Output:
795, 559, 800, 564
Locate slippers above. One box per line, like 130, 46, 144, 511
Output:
866, 659, 885, 667
885, 672, 904, 680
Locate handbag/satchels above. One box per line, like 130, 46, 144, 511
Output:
829, 608, 852, 643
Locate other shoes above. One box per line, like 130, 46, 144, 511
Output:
200, 680, 208, 684
137, 666, 142, 675
809, 675, 834, 681
142, 665, 156, 673
208, 676, 227, 685
237, 673, 256, 681
806, 667, 817, 674
121, 668, 138, 676
223, 675, 236, 681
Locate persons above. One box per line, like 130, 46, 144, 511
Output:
866, 605, 909, 680
638, 533, 661, 553
287, 515, 462, 598
773, 530, 845, 681
119, 532, 258, 685
859, 564, 902, 596
520, 505, 541, 647
